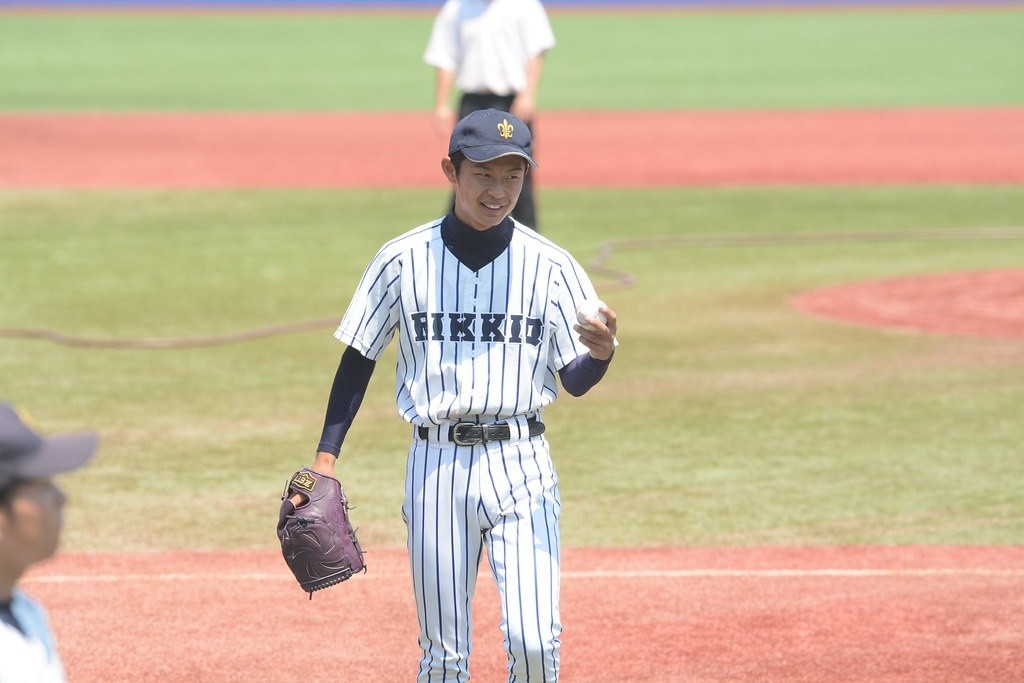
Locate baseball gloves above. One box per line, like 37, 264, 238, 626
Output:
275, 465, 366, 596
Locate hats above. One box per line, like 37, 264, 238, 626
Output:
0, 402, 96, 485
448, 106, 538, 169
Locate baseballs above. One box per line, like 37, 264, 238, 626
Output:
576, 296, 608, 328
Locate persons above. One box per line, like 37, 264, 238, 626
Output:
422, 0, 556, 233
277, 109, 618, 683
0, 408, 99, 683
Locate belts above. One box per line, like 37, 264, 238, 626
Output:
418, 415, 546, 445
